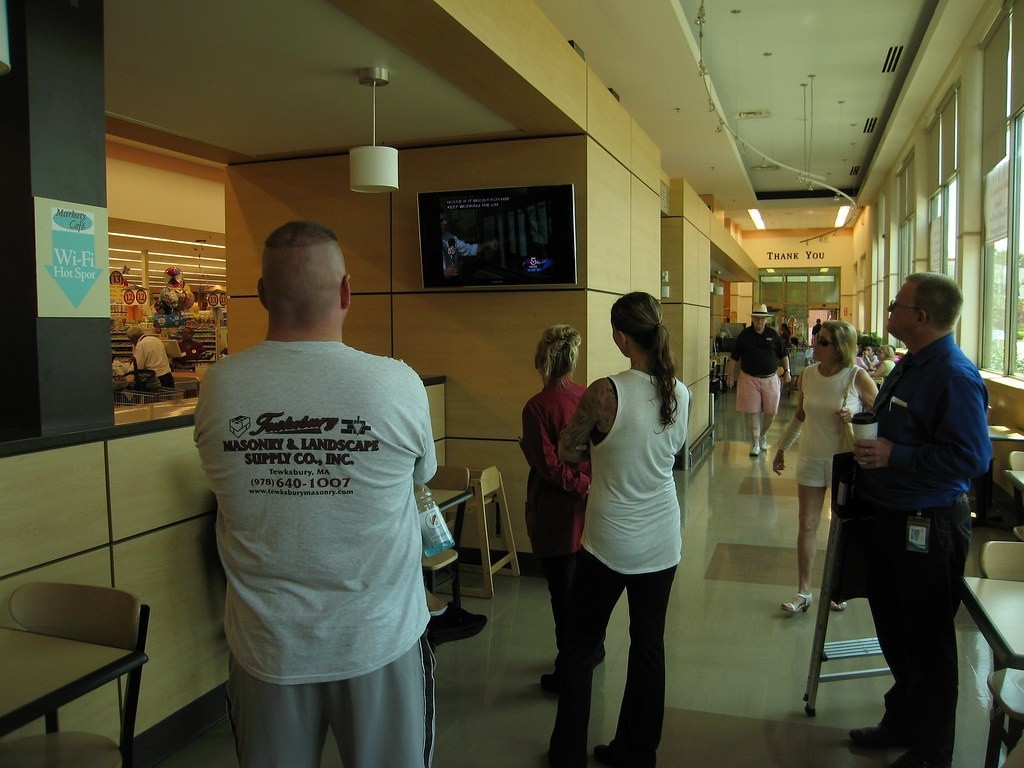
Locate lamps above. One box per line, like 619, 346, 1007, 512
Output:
349, 67, 399, 194
715, 270, 724, 295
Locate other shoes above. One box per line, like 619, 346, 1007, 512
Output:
850, 722, 908, 747
540, 673, 562, 702
594, 742, 657, 768
893, 746, 954, 768
595, 645, 607, 669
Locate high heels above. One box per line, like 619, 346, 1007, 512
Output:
782, 593, 814, 613
831, 598, 845, 612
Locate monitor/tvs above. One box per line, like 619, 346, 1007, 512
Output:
417, 184, 577, 288
161, 340, 181, 358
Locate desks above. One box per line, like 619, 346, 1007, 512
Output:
430, 489, 473, 513
960, 576, 1024, 665
971, 425, 1024, 531
0, 626, 149, 735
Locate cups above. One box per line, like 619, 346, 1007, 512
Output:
850, 412, 878, 465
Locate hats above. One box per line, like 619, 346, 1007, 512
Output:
750, 303, 774, 316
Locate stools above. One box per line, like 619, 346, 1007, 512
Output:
435, 465, 521, 598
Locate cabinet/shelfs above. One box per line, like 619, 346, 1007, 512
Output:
111, 319, 227, 361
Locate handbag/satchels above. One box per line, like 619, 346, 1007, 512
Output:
838, 367, 859, 454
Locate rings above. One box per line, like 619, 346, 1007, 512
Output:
865, 449, 867, 461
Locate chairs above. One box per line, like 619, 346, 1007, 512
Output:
709, 352, 731, 402
980, 451, 1024, 768
0, 582, 149, 768
425, 466, 471, 606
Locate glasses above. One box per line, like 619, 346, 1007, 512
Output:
816, 333, 838, 347
755, 317, 767, 321
890, 298, 931, 322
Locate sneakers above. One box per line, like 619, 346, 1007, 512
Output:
760, 434, 768, 451
750, 441, 760, 455
429, 599, 487, 646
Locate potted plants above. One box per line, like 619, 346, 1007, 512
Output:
858, 331, 882, 355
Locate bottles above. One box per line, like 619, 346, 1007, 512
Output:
415, 480, 455, 557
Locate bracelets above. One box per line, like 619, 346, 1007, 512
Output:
785, 369, 790, 373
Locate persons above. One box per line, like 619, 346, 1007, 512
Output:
127, 326, 175, 388
194, 221, 487, 768
440, 208, 499, 280
849, 273, 992, 768
549, 292, 694, 768
773, 320, 879, 614
780, 323, 792, 347
855, 345, 900, 379
812, 319, 823, 336
180, 326, 205, 360
520, 324, 606, 700
726, 303, 792, 456
